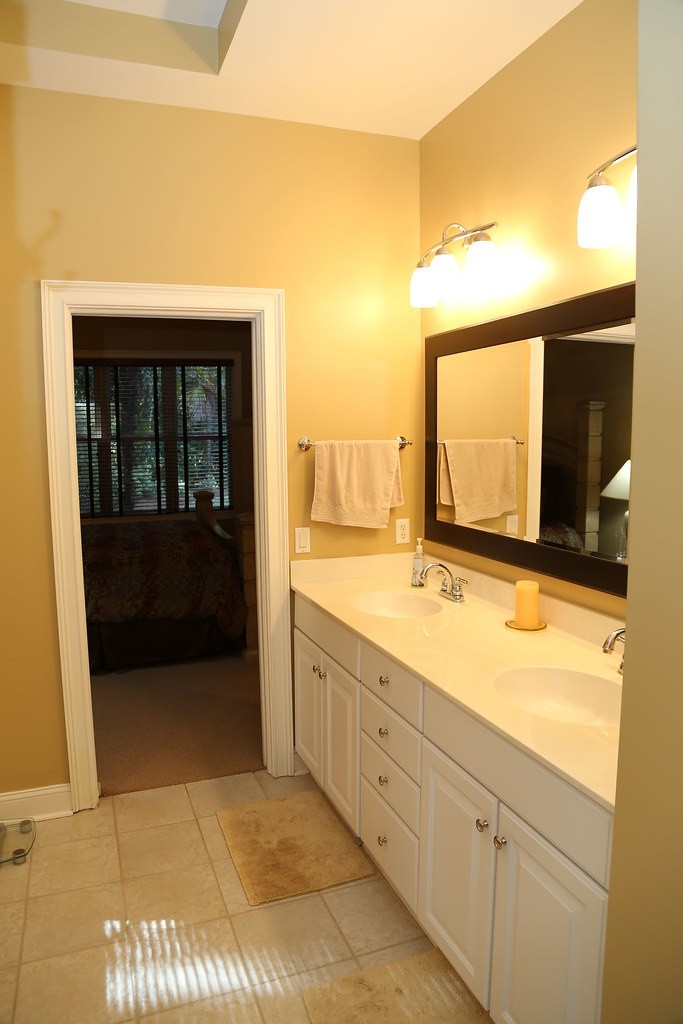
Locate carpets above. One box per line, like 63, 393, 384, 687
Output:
301, 948, 494, 1024
215, 789, 377, 907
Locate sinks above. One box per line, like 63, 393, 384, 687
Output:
350, 587, 445, 622
493, 661, 624, 728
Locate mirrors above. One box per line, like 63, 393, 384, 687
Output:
424, 278, 636, 599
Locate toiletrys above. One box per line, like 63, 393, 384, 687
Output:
407, 536, 427, 586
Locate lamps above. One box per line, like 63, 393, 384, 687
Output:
408, 221, 519, 311
576, 143, 637, 250
600, 458, 632, 566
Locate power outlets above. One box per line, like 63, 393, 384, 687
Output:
395, 518, 410, 545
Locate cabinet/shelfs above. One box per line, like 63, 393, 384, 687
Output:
358, 636, 425, 933
418, 680, 609, 1024
293, 593, 363, 842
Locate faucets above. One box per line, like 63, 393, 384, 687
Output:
601, 627, 625, 675
420, 561, 457, 595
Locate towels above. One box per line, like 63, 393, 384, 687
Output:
440, 436, 520, 526
308, 438, 406, 531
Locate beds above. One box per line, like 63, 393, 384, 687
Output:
540, 398, 607, 553
80, 489, 259, 676
